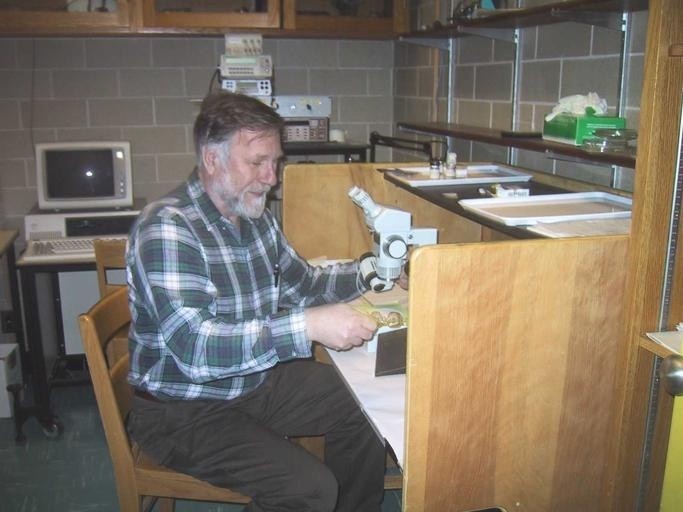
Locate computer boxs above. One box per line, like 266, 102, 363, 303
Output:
23, 197, 147, 242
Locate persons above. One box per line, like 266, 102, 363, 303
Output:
123, 91, 410, 511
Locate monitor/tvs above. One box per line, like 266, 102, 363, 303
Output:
34, 140, 134, 210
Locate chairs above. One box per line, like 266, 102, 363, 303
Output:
78, 283, 252, 510
94, 238, 128, 343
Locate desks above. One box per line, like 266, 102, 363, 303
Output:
12, 246, 127, 447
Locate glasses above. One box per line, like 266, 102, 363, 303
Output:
370, 311, 403, 328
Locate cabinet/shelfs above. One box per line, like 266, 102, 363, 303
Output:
278, 160, 637, 511
397, 1, 645, 169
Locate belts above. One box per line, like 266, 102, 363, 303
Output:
135, 388, 160, 402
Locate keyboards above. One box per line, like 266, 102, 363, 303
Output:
21, 233, 129, 260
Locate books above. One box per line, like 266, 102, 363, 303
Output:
374, 327, 405, 376
644, 330, 682, 357
